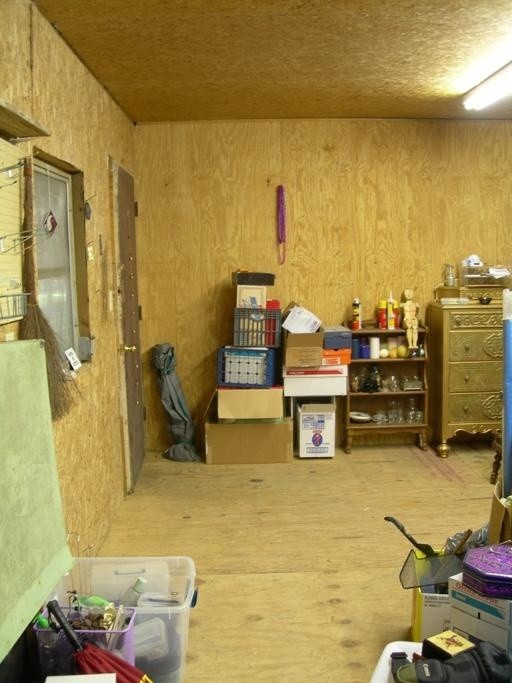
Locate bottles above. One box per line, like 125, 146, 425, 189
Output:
352, 297, 362, 329
112, 577, 148, 624
377, 299, 399, 329
351, 338, 360, 358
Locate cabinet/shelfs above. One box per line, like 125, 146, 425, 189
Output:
342, 319, 430, 453
425, 300, 504, 458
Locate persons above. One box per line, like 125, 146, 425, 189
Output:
399, 289, 420, 351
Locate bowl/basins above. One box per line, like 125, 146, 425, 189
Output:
478, 297, 491, 304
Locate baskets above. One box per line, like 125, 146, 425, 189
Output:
217, 308, 282, 388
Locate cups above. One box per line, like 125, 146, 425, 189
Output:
360, 345, 369, 358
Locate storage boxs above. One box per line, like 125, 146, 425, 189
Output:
32, 555, 198, 683
281, 301, 353, 459
200, 385, 294, 465
369, 547, 512, 683
